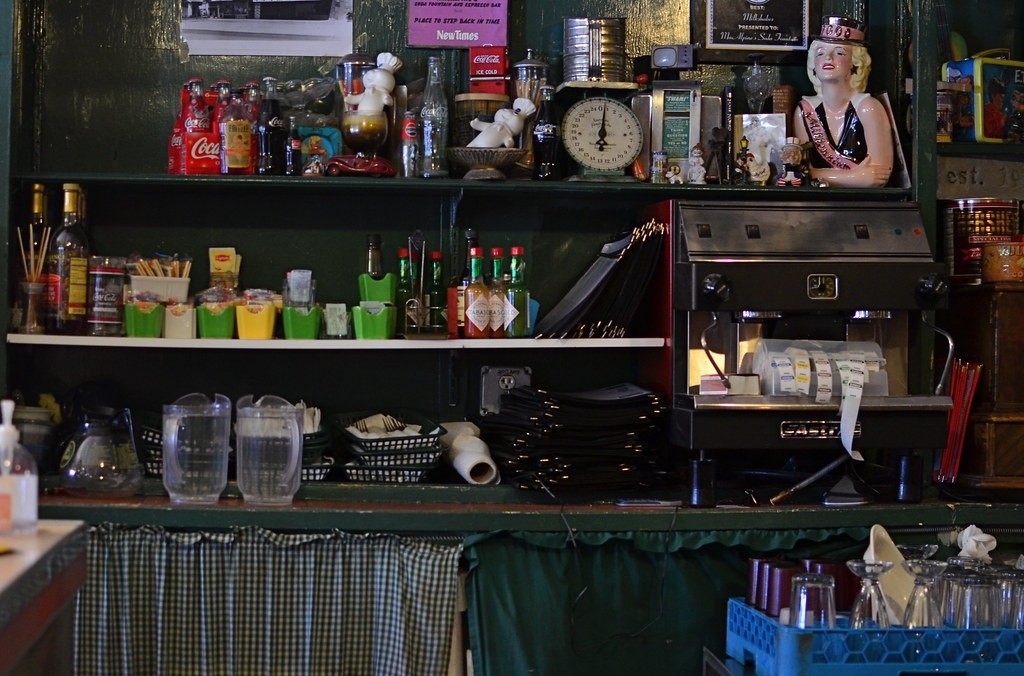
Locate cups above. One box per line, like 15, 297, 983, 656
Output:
939, 558, 1024, 629
747, 555, 860, 616
531, 85, 561, 181
339, 110, 388, 158
790, 574, 836, 631
124, 273, 397, 339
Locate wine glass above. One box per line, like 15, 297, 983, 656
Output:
847, 543, 949, 629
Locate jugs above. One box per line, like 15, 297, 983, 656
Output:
60, 401, 146, 499
234, 395, 303, 506
161, 393, 232, 505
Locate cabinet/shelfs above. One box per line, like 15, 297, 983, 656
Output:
8, 1, 935, 503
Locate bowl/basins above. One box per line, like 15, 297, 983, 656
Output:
130, 276, 191, 302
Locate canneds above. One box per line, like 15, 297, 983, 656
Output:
909, 92, 955, 142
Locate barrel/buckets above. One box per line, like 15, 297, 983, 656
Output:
937, 194, 1024, 289
12, 407, 56, 449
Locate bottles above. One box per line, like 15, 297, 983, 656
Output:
365, 233, 384, 281
425, 251, 447, 333
489, 248, 504, 339
169, 78, 261, 175
48, 183, 92, 335
504, 247, 530, 338
336, 46, 376, 155
456, 229, 478, 338
2, 424, 40, 534
419, 57, 450, 178
256, 77, 301, 176
503, 274, 512, 286
20, 183, 49, 333
90, 255, 126, 336
398, 248, 425, 333
464, 247, 489, 338
403, 110, 418, 179
652, 151, 667, 184
513, 49, 548, 174
447, 267, 456, 335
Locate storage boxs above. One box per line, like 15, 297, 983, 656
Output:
727, 596, 1024, 676
135, 406, 448, 485
